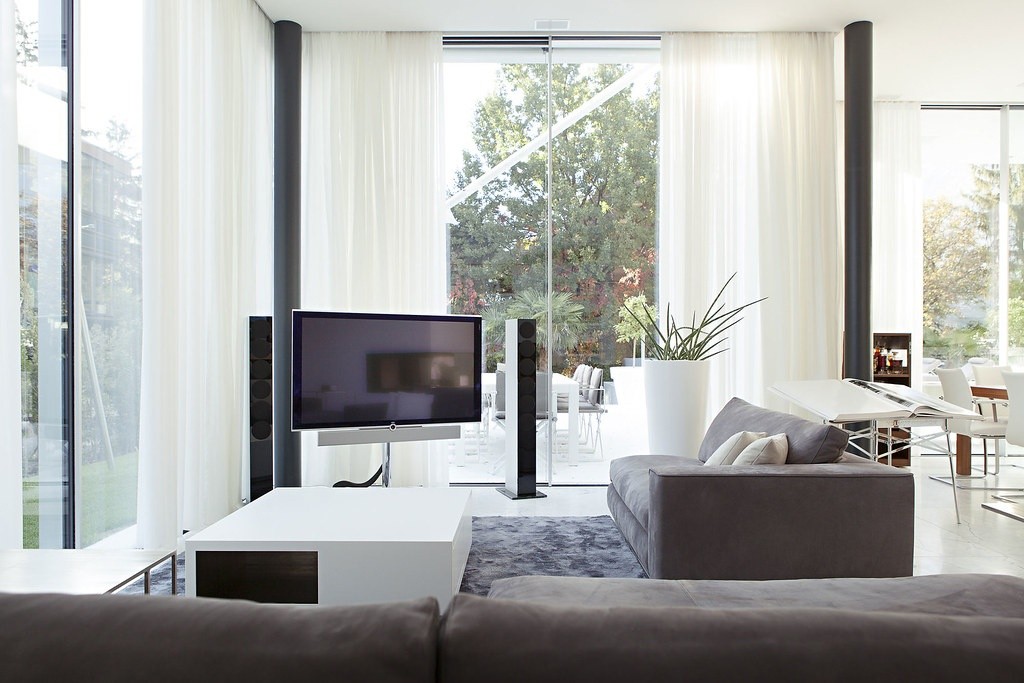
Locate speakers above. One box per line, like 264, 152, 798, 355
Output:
245, 315, 274, 503
495, 318, 547, 500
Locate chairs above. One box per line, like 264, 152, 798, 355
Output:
971, 365, 1024, 476
982, 369, 1024, 522
554, 364, 586, 441
555, 365, 595, 451
554, 367, 608, 463
929, 368, 1024, 490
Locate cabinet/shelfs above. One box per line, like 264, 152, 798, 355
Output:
839, 332, 911, 467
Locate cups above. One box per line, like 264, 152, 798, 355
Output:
889, 359, 903, 374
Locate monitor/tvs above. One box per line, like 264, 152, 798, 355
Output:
291, 309, 482, 432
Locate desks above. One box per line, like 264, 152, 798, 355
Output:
482, 373, 580, 465
0, 548, 177, 595
767, 376, 987, 525
956, 384, 1009, 476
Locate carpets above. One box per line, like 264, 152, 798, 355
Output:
460, 514, 648, 598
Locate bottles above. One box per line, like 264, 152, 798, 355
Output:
873, 344, 887, 374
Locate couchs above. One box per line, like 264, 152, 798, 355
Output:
0, 591, 438, 683
607, 397, 914, 581
438, 573, 1024, 683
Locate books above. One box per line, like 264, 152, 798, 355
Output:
769, 377, 984, 422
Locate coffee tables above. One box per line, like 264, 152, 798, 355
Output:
185, 486, 472, 614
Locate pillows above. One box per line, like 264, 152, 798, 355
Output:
733, 433, 789, 466
704, 430, 768, 465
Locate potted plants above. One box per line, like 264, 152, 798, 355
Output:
621, 270, 769, 458
